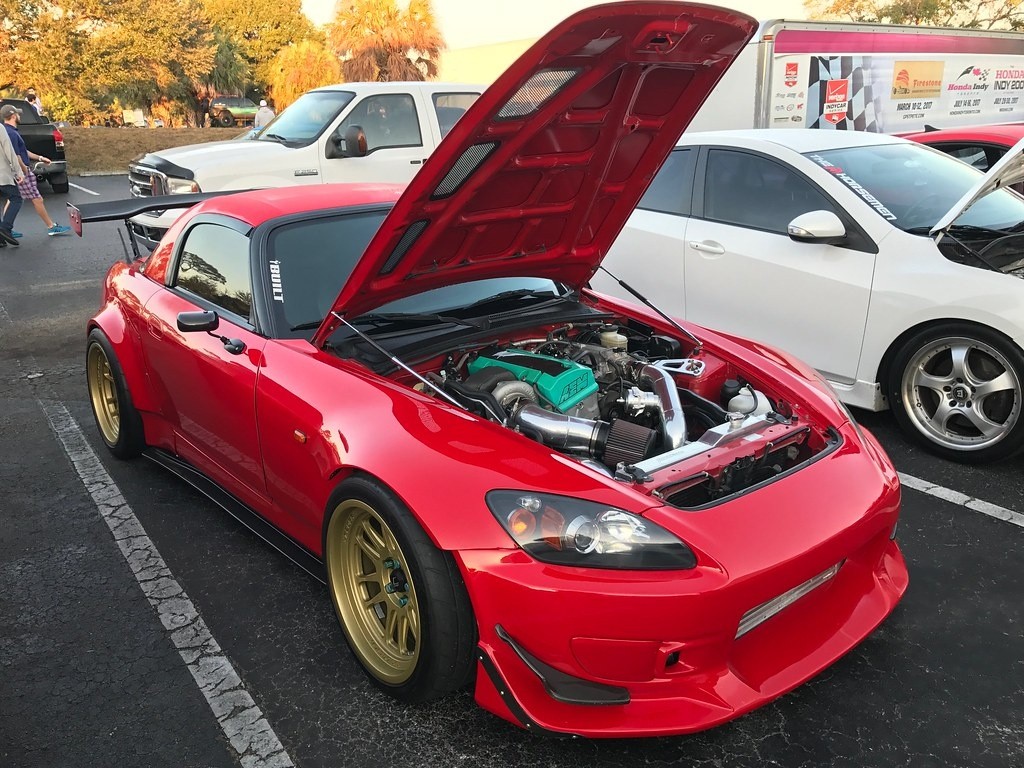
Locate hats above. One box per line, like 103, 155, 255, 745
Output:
0, 104, 22, 118
260, 100, 267, 107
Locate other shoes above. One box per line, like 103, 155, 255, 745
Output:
0, 226, 20, 246
0, 238, 7, 247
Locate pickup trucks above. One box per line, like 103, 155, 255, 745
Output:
0, 98, 70, 194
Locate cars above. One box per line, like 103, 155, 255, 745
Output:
580, 127, 1024, 470
83, 0, 909, 741
853, 123, 1024, 228
153, 118, 165, 128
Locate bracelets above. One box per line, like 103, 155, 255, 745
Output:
39, 156, 43, 162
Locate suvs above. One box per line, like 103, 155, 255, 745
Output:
208, 94, 276, 129
128, 80, 517, 252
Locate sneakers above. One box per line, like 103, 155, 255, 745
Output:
47, 222, 72, 235
11, 229, 23, 238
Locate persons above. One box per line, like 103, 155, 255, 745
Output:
194, 93, 210, 128
89, 118, 111, 127
0, 105, 72, 247
255, 99, 275, 129
25, 87, 46, 117
